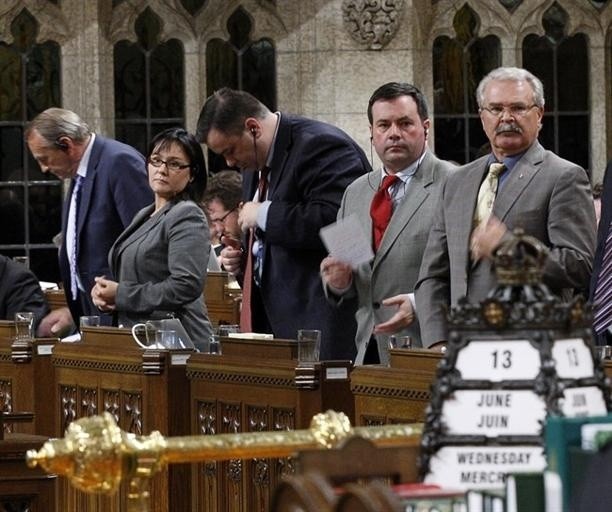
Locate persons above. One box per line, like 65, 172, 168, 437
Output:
0, 251, 48, 336
88, 128, 218, 354
418, 69, 599, 361
202, 169, 249, 243
195, 88, 383, 365
25, 108, 158, 339
318, 81, 466, 366
586, 159, 610, 360
206, 222, 226, 272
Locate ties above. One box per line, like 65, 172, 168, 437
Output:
71, 176, 83, 300
239, 166, 271, 333
370, 176, 400, 255
471, 162, 506, 260
592, 218, 612, 335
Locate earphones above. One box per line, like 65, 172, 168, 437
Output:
57, 143, 68, 148
191, 164, 200, 177
370, 129, 373, 140
424, 126, 427, 135
250, 127, 257, 136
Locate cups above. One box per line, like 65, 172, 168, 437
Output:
13, 256, 29, 270
220, 324, 239, 336
158, 330, 177, 350
297, 328, 321, 362
80, 316, 101, 337
146, 319, 165, 348
208, 334, 221, 355
14, 312, 36, 341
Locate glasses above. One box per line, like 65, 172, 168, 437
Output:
211, 206, 237, 226
148, 158, 192, 171
481, 104, 539, 115
